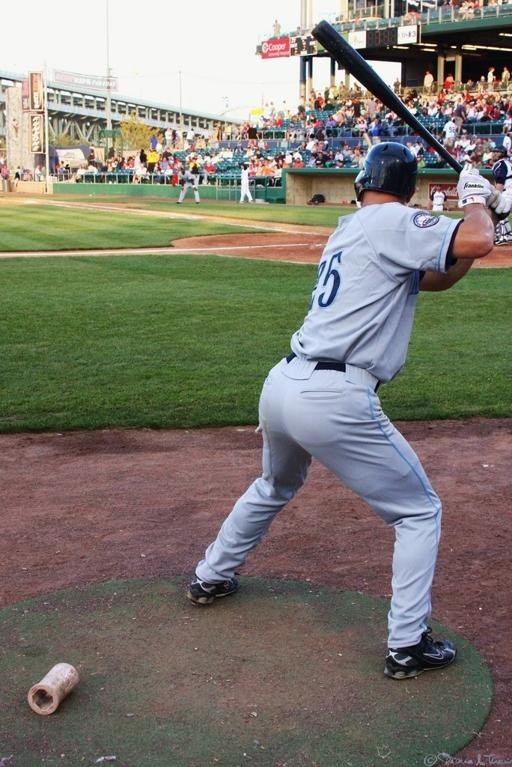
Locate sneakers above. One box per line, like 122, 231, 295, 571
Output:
384, 628, 456, 679
494, 233, 512, 246
187, 571, 240, 605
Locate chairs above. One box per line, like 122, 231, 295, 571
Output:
69, 119, 282, 187
263, 0, 511, 42
283, 78, 511, 170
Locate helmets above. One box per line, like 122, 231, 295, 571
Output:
493, 146, 507, 161
353, 143, 417, 201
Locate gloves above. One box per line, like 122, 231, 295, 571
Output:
457, 160, 491, 208
487, 189, 511, 221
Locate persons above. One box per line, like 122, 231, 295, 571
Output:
1, 0, 511, 205
188, 142, 510, 679
490, 145, 511, 245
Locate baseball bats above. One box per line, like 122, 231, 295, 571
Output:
311, 20, 511, 221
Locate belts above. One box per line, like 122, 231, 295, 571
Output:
286, 351, 345, 373
